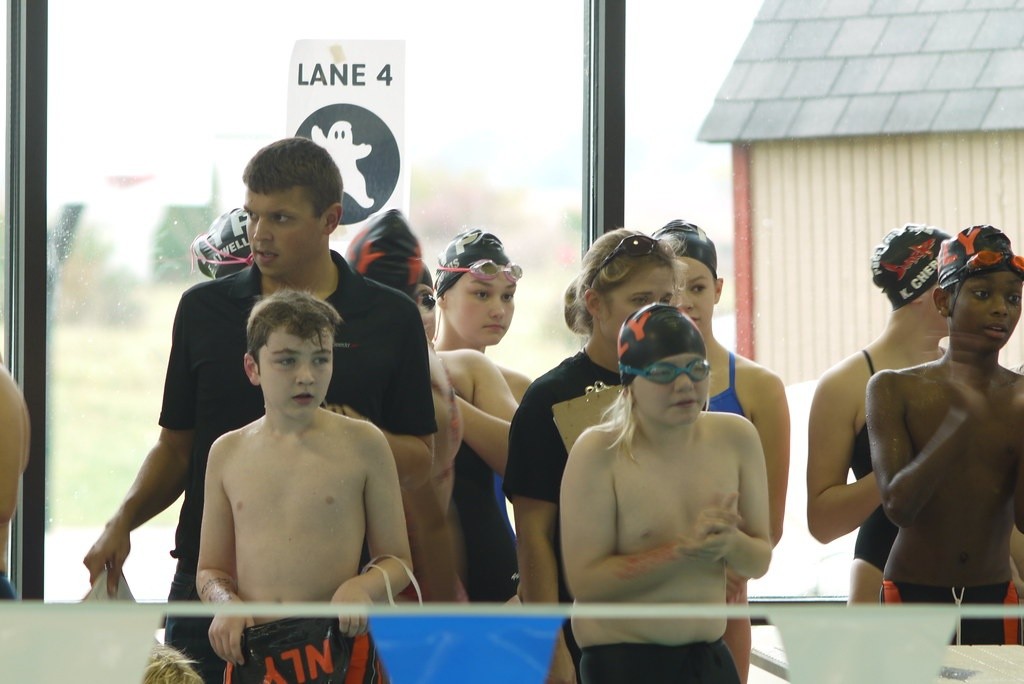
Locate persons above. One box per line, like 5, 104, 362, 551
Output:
196, 292, 414, 684
415, 264, 520, 605
0, 355, 29, 602
347, 208, 460, 605
865, 226, 1024, 645
559, 303, 772, 684
84, 137, 438, 684
190, 209, 257, 280
435, 229, 533, 549
651, 219, 791, 684
807, 224, 952, 605
502, 230, 682, 684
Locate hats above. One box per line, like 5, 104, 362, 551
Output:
414, 261, 432, 287
342, 208, 422, 295
650, 219, 718, 278
617, 302, 706, 384
870, 223, 948, 311
938, 225, 1022, 276
435, 230, 514, 294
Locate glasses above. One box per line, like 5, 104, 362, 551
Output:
590, 235, 675, 280
947, 249, 1024, 281
618, 358, 711, 385
437, 258, 525, 283
416, 294, 436, 311
